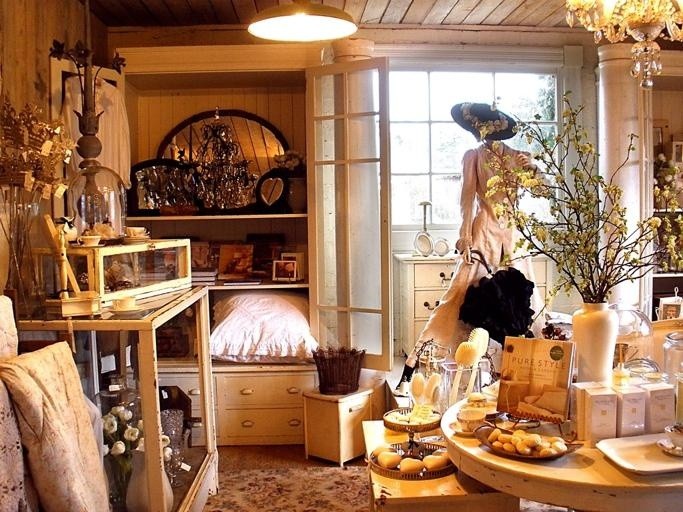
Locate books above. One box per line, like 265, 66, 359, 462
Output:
497, 334, 577, 425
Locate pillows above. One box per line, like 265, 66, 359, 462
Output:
0, 340, 116, 512
208, 292, 320, 365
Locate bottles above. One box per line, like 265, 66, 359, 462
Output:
412, 343, 497, 414
662, 330, 683, 430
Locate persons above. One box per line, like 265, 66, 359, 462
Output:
663, 305, 677, 320
396, 101, 555, 390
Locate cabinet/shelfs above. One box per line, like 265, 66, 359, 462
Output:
394, 252, 554, 361
652, 51, 683, 321
302, 387, 377, 467
215, 373, 317, 447
49, 43, 395, 372
108, 373, 215, 448
13, 286, 220, 512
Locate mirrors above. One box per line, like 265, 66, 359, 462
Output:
127, 159, 205, 213
156, 107, 287, 176
256, 168, 288, 214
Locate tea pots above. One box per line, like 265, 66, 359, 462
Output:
55, 217, 78, 243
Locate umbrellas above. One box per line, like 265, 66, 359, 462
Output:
458, 250, 536, 350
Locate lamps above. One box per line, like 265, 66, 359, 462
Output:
566, 0, 683, 89
247, 0, 358, 42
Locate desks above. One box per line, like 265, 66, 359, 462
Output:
360, 419, 521, 512
439, 387, 683, 512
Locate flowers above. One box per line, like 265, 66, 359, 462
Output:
472, 90, 683, 326
100, 396, 174, 462
272, 149, 311, 178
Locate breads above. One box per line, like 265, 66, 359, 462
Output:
487, 427, 568, 457
371, 446, 448, 474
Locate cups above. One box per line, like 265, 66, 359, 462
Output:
126, 226, 146, 238
77, 235, 101, 246
112, 295, 137, 311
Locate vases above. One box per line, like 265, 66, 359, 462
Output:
125, 450, 174, 512
4, 203, 38, 316
288, 177, 307, 214
571, 302, 619, 383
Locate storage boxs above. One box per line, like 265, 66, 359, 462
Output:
33, 239, 191, 309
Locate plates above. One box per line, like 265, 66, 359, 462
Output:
413, 231, 434, 258
108, 308, 141, 316
449, 402, 683, 482
433, 237, 451, 257
123, 236, 151, 244
72, 244, 105, 249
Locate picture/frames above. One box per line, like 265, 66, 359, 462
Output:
189, 231, 287, 282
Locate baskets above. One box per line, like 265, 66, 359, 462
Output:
312, 344, 367, 394
